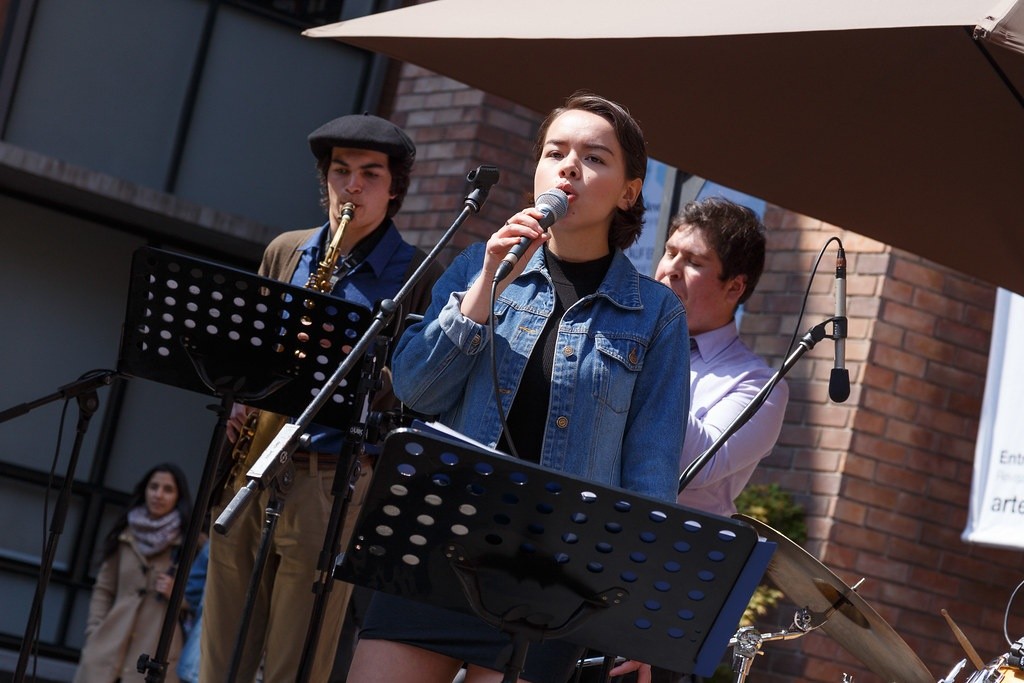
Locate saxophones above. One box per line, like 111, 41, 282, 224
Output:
228, 200, 357, 496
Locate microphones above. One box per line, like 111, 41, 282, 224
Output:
494, 187, 570, 285
828, 249, 850, 403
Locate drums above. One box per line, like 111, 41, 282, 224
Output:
964, 652, 1024, 683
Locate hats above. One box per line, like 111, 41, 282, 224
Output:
308, 111, 416, 160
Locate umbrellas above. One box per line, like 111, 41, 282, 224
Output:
301, 0, 1024, 295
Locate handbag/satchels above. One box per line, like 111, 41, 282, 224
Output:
178, 607, 194, 646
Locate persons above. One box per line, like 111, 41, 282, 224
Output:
177, 543, 210, 683
609, 200, 791, 682
75, 462, 195, 683
346, 97, 691, 683
198, 115, 445, 683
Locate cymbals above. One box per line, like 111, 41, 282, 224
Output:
729, 512, 938, 683
